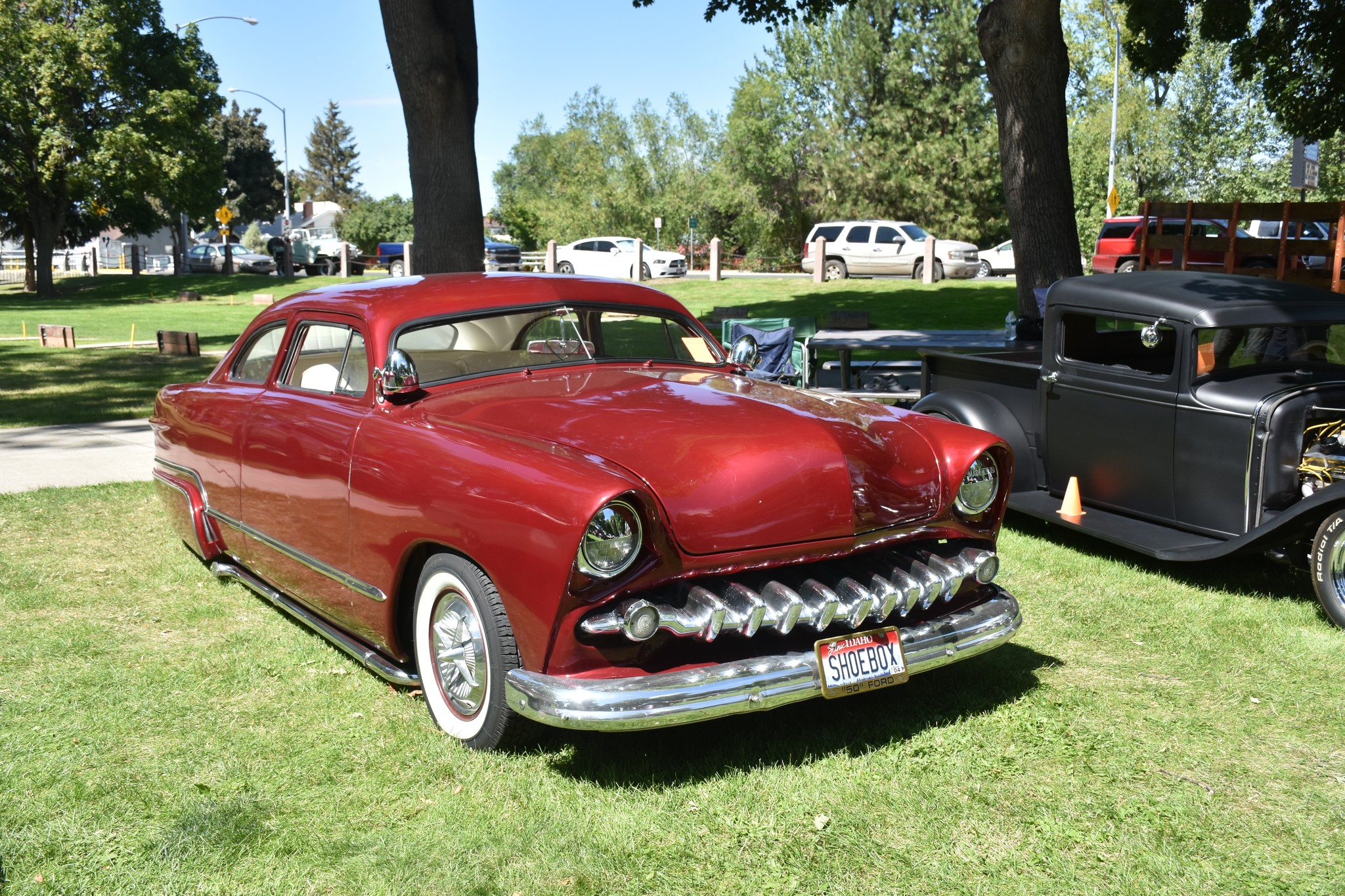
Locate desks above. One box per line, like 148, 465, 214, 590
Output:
806, 328, 1018, 390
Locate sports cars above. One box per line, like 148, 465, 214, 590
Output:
147, 273, 1023, 752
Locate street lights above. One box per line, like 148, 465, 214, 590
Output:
227, 88, 295, 276
175, 16, 260, 277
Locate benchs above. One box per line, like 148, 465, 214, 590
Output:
812, 362, 921, 408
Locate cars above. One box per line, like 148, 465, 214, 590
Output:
543, 237, 687, 281
975, 239, 1087, 278
182, 242, 277, 275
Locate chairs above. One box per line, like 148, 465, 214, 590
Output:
288, 349, 588, 391
719, 317, 818, 392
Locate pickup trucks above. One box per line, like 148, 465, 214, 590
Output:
910, 269, 1345, 634
376, 236, 523, 278
1205, 219, 1345, 280
272, 228, 365, 276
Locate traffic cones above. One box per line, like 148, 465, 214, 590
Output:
1056, 476, 1086, 516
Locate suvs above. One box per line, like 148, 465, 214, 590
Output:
1092, 215, 1306, 274
801, 220, 982, 283
